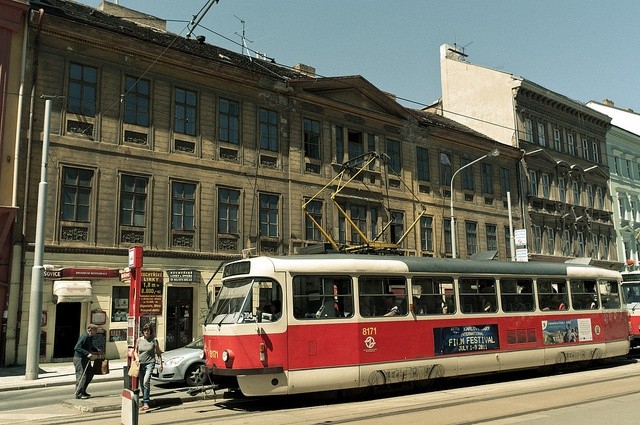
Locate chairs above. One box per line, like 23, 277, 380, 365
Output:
368, 306, 375, 316
461, 303, 473, 313
419, 305, 427, 315
573, 302, 582, 309
504, 303, 513, 312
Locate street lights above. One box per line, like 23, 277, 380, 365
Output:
450, 149, 502, 262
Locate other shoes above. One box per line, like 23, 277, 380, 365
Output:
141, 405, 149, 411
74, 392, 91, 398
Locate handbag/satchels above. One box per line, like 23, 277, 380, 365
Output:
128, 351, 141, 378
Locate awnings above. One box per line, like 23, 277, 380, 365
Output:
53, 281, 92, 304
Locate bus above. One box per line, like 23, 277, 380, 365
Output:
203, 253, 640, 402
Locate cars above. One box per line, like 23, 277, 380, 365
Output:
151, 336, 209, 386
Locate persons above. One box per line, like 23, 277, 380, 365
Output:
382, 291, 403, 316
433, 294, 448, 314
480, 295, 493, 313
134, 322, 163, 410
553, 295, 566, 310
563, 323, 579, 342
73, 324, 103, 398
585, 294, 597, 309
413, 296, 424, 316
628, 286, 636, 302
